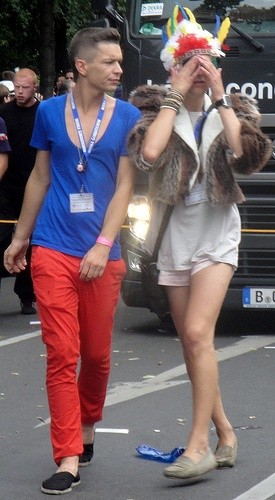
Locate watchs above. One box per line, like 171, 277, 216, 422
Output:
215, 94, 233, 107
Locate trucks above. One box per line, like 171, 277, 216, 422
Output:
103, 0, 275, 326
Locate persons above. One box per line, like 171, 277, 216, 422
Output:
4, 27, 143, 495
129, 4, 272, 479
0, 68, 77, 315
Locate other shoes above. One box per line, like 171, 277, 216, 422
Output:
41, 471, 81, 494
20, 298, 36, 314
78, 428, 95, 466
214, 438, 238, 467
163, 448, 218, 479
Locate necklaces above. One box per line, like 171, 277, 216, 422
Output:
72, 118, 84, 172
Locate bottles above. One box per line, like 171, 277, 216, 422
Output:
136, 444, 185, 463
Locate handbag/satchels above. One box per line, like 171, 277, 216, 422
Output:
141, 256, 168, 314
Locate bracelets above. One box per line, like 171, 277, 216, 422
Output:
159, 88, 184, 115
96, 236, 113, 247
